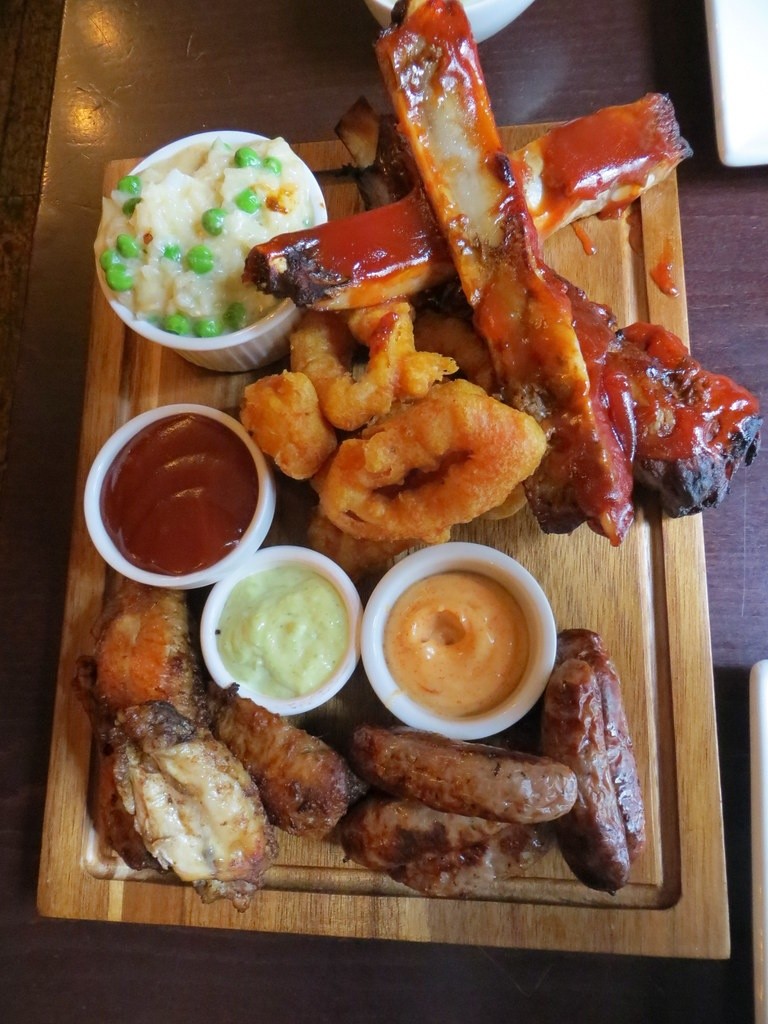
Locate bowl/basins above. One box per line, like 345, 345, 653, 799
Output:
361, 542, 558, 743
82, 403, 278, 591
200, 545, 363, 716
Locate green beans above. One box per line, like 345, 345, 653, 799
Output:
98, 146, 284, 339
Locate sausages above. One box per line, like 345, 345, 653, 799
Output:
332, 625, 644, 895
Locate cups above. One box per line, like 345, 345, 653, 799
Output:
95, 130, 332, 372
